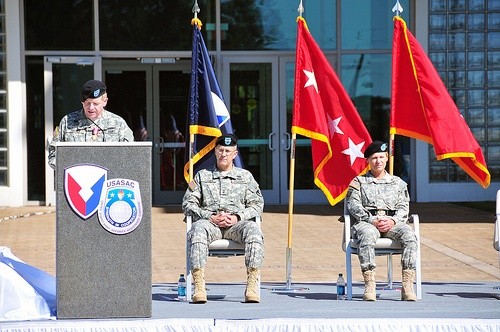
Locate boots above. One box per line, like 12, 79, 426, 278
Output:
245, 267, 261, 303
363, 270, 377, 301
191, 266, 208, 303
401, 268, 416, 301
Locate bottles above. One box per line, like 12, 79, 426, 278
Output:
178, 273, 186, 302
336, 274, 345, 300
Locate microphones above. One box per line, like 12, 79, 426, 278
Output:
74, 117, 106, 142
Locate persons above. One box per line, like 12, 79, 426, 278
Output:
182, 135, 264, 304
48, 80, 134, 170
345, 140, 418, 302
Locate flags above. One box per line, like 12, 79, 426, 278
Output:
290, 16, 375, 207
389, 15, 491, 189
138, 116, 186, 166
183, 19, 242, 186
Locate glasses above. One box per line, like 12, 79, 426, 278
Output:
215, 148, 237, 155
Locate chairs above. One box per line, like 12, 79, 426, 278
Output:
186, 211, 259, 302
342, 197, 422, 300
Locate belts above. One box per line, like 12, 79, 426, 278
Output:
368, 210, 395, 217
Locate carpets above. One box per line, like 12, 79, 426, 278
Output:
152, 281, 498, 318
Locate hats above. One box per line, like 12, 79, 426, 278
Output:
80, 79, 106, 101
365, 141, 388, 157
216, 133, 238, 146
